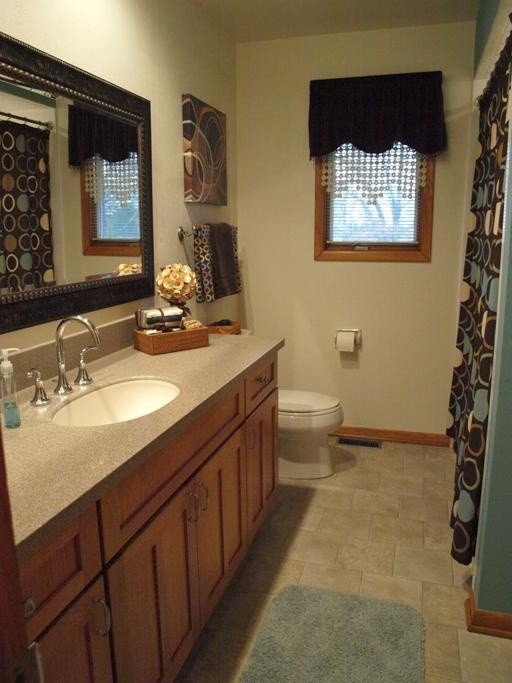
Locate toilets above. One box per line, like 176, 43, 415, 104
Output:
239, 330, 343, 478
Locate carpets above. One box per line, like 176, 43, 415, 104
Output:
237, 585, 427, 683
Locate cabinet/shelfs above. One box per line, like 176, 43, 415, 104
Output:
18, 351, 280, 683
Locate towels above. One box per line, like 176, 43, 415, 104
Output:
193, 223, 242, 304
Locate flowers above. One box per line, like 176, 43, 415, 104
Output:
111, 263, 141, 277
154, 262, 201, 317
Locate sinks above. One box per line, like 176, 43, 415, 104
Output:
47, 375, 181, 427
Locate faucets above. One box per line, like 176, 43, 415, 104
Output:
52, 317, 101, 394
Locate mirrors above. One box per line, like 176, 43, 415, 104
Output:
0, 31, 155, 335
